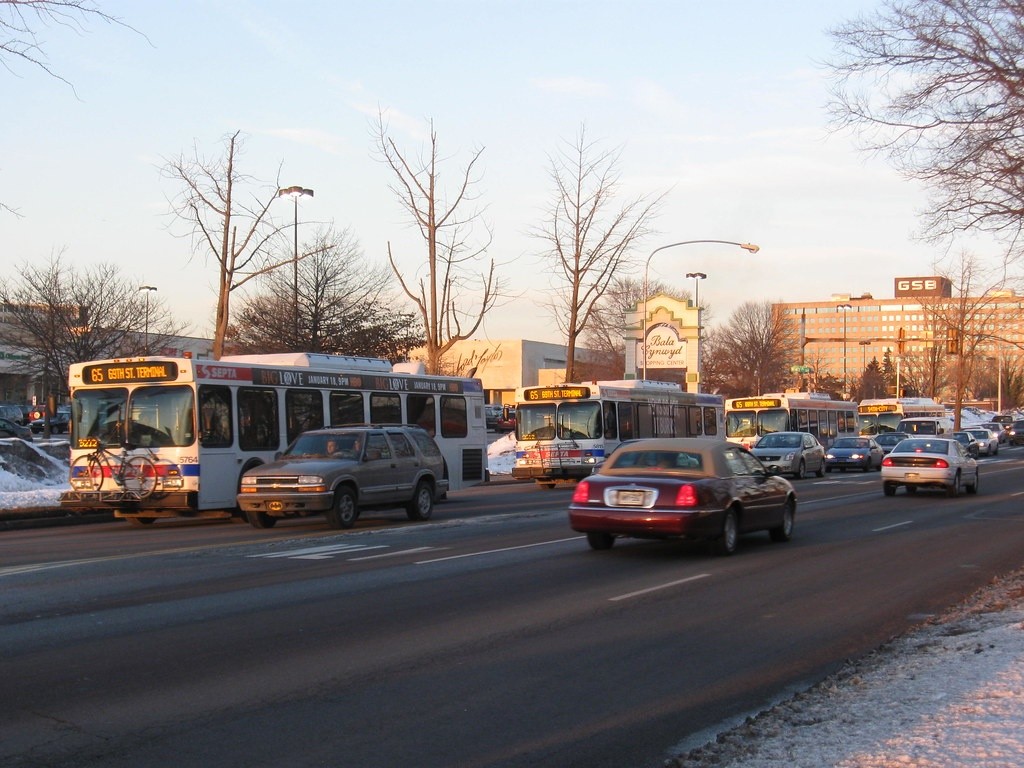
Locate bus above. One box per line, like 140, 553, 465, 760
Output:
58, 350, 489, 535
511, 380, 737, 490
860, 398, 946, 435
725, 391, 859, 452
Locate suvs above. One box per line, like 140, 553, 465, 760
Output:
235, 422, 452, 528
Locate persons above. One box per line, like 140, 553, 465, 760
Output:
325, 436, 340, 456
349, 436, 369, 458
856, 439, 868, 448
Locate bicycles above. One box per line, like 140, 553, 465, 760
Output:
68, 431, 160, 502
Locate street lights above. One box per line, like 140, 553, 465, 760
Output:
859, 340, 873, 373
900, 328, 906, 353
837, 304, 852, 394
642, 238, 761, 382
686, 272, 708, 309
986, 356, 998, 410
139, 285, 159, 357
279, 185, 314, 351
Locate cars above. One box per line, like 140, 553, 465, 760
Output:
0, 402, 73, 442
748, 431, 827, 480
484, 404, 516, 432
825, 415, 1024, 498
569, 440, 798, 557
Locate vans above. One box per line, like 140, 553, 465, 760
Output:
897, 417, 962, 442
